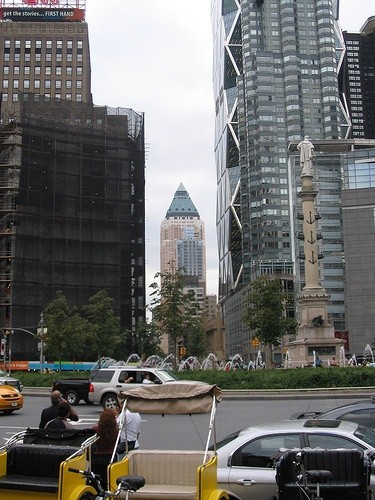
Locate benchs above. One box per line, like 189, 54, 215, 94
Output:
275, 448, 373, 500
0, 444, 81, 493
106, 450, 217, 500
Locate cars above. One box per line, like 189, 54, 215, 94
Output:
290, 394, 374, 430
0, 382, 24, 414
208, 418, 374, 500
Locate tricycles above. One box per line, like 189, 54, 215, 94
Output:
68, 380, 231, 500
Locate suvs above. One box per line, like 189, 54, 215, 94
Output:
0, 370, 23, 392
89, 366, 181, 413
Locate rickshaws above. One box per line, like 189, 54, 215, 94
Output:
0, 427, 102, 500
274, 447, 372, 500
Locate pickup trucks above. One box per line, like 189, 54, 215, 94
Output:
52, 367, 93, 407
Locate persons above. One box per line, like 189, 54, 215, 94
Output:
226, 363, 239, 372
315, 357, 340, 366
39, 391, 141, 454
124, 375, 155, 384
296, 135, 315, 175
